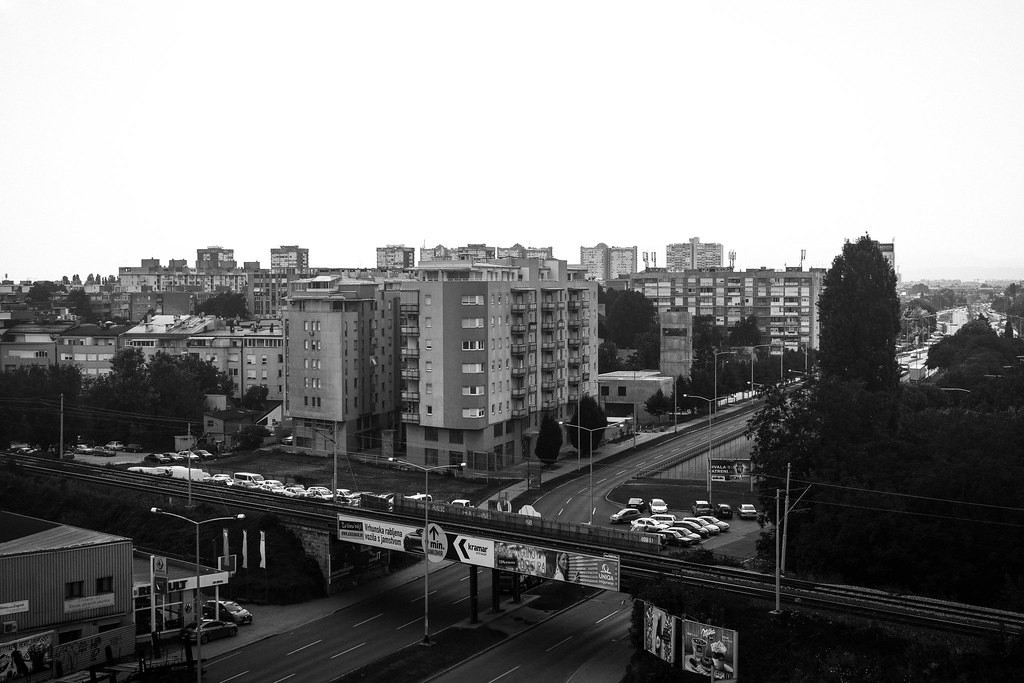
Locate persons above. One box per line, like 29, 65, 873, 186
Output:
10, 643, 31, 678
552, 552, 581, 584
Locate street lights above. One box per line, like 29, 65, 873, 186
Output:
387, 457, 468, 646
750, 344, 775, 407
558, 421, 625, 526
780, 508, 812, 575
633, 368, 653, 449
674, 358, 700, 437
151, 506, 246, 683
577, 380, 607, 472
309, 427, 338, 503
714, 352, 738, 422
780, 337, 797, 388
683, 393, 738, 506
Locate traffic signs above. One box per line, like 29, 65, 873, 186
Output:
421, 523, 449, 563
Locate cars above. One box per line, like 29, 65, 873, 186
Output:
608, 495, 763, 551
180, 616, 239, 645
7, 435, 475, 518
404, 527, 425, 555
202, 597, 254, 626
901, 362, 909, 371
497, 570, 543, 596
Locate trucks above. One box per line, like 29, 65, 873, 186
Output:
909, 364, 926, 381
